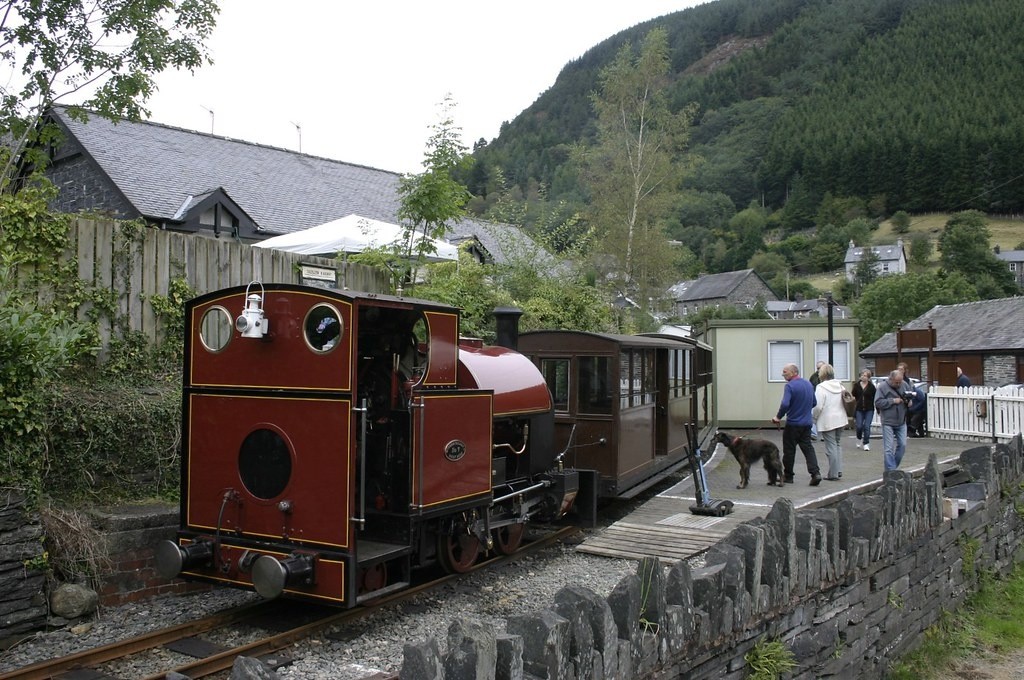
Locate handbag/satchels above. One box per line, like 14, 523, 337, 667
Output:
840, 383, 856, 417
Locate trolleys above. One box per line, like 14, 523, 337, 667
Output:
682, 423, 734, 517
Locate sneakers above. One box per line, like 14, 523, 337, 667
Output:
864, 444, 869, 451
856, 438, 861, 448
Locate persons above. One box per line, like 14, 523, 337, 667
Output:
771, 363, 823, 486
874, 362, 926, 471
957, 366, 971, 388
851, 368, 881, 451
808, 360, 849, 481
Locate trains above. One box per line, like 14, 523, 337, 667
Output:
156, 281, 714, 613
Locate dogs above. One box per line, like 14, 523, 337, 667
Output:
710, 432, 784, 489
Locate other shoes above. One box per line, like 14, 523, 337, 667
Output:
811, 435, 817, 440
809, 474, 822, 485
823, 472, 842, 480
777, 476, 794, 483
821, 437, 824, 441
